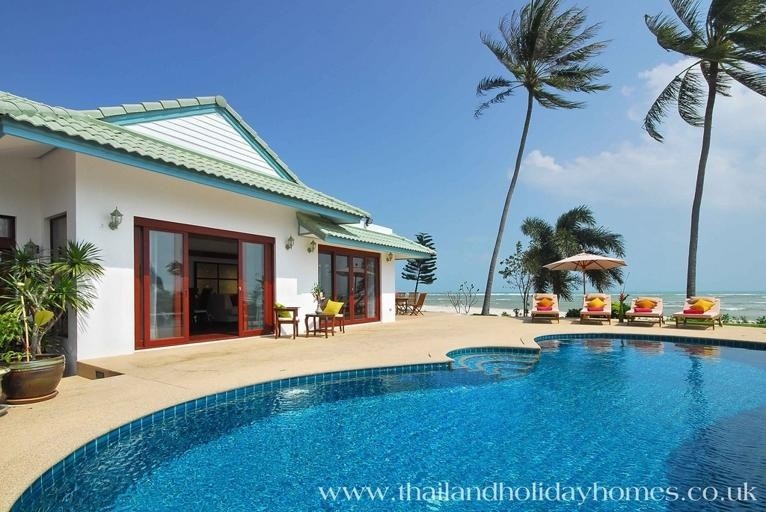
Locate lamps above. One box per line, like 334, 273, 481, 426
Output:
107, 205, 123, 230
386, 252, 394, 262
20, 237, 39, 261
284, 232, 295, 251
307, 240, 317, 254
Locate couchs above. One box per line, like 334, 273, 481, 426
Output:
205, 291, 242, 324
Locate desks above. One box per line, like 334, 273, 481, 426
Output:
304, 312, 337, 338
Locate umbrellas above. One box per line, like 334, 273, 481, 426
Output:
329, 263, 375, 292
542, 249, 628, 296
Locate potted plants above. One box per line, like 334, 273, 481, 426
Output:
0, 234, 107, 406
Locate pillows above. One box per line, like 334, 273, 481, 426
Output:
534, 294, 556, 313
322, 300, 345, 315
273, 303, 291, 319
682, 296, 719, 317
585, 294, 607, 314
631, 296, 659, 316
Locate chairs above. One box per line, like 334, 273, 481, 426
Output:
579, 291, 615, 326
624, 295, 667, 329
671, 294, 725, 333
273, 306, 300, 341
530, 291, 561, 326
319, 296, 348, 336
193, 287, 213, 329
394, 290, 427, 317
